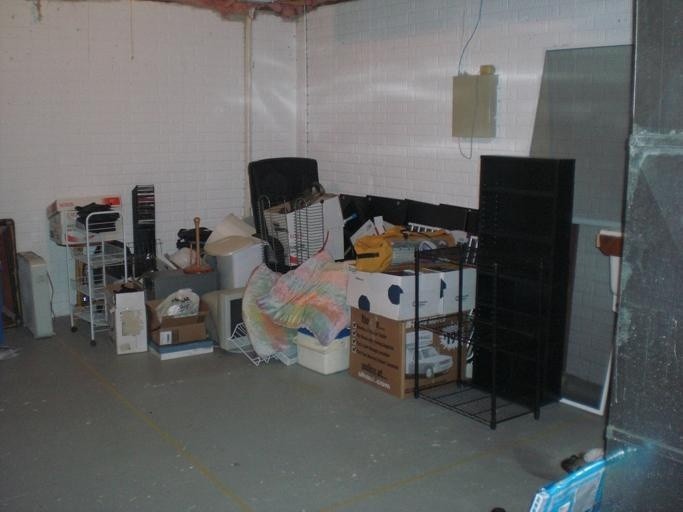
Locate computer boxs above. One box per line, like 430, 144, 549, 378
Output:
143, 270, 216, 299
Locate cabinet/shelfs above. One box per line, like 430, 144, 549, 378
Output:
414, 243, 553, 429
65, 210, 127, 346
258, 194, 324, 273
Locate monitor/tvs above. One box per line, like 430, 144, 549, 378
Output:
200, 287, 255, 354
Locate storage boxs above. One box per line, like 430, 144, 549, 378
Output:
350, 307, 473, 399
346, 267, 476, 322
293, 328, 350, 375
46, 192, 125, 247
264, 195, 344, 266
107, 291, 148, 355
145, 299, 209, 346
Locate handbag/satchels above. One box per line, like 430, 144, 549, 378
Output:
352, 225, 455, 272
